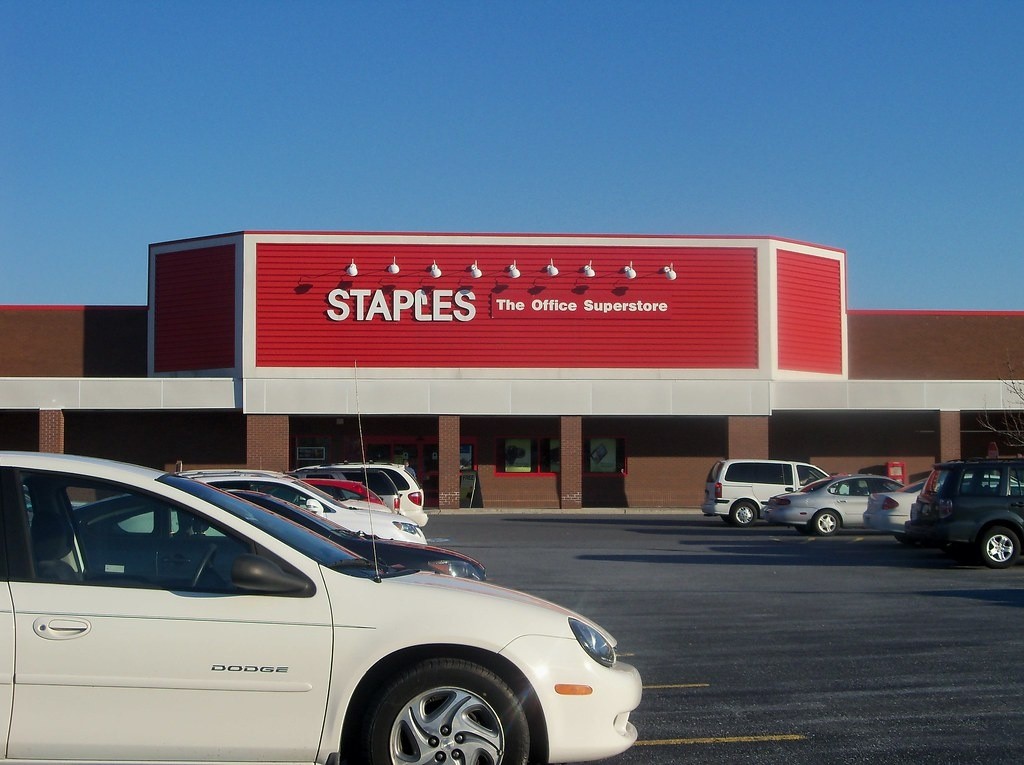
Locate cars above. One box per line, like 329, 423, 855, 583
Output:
286, 467, 400, 517
862, 474, 1023, 547
27, 486, 488, 592
183, 475, 428, 546
300, 477, 386, 507
792, 473, 851, 494
0, 448, 644, 765
760, 473, 907, 536
175, 469, 392, 514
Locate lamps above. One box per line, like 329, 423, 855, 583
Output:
388, 257, 399, 274
347, 259, 358, 277
546, 257, 559, 276
508, 259, 521, 278
664, 262, 676, 280
623, 261, 636, 279
431, 260, 442, 278
584, 260, 595, 277
471, 260, 482, 279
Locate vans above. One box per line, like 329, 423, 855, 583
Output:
699, 459, 831, 528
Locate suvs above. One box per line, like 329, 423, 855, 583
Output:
903, 456, 1024, 568
287, 461, 430, 527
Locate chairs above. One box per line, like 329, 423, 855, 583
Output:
170, 510, 209, 538
29, 509, 82, 586
